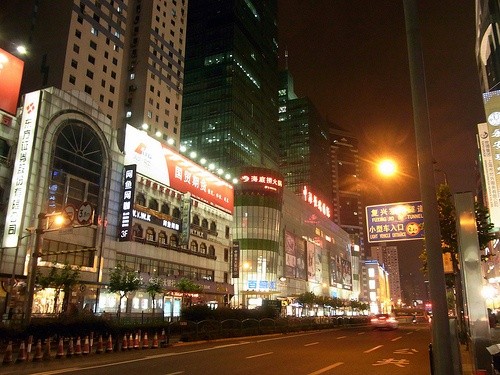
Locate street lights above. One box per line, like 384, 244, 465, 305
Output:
372, 159, 467, 344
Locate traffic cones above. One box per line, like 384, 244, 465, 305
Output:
2, 341, 14, 366
32, 338, 43, 361
73, 336, 83, 357
82, 335, 91, 356
134, 333, 140, 351
54, 337, 65, 359
151, 333, 160, 349
142, 332, 150, 350
15, 341, 26, 363
42, 337, 52, 360
95, 335, 105, 355
66, 337, 75, 359
105, 334, 114, 353
120, 334, 127, 352
128, 333, 135, 352
160, 329, 167, 348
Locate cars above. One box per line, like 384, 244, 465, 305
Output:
371, 314, 399, 329
